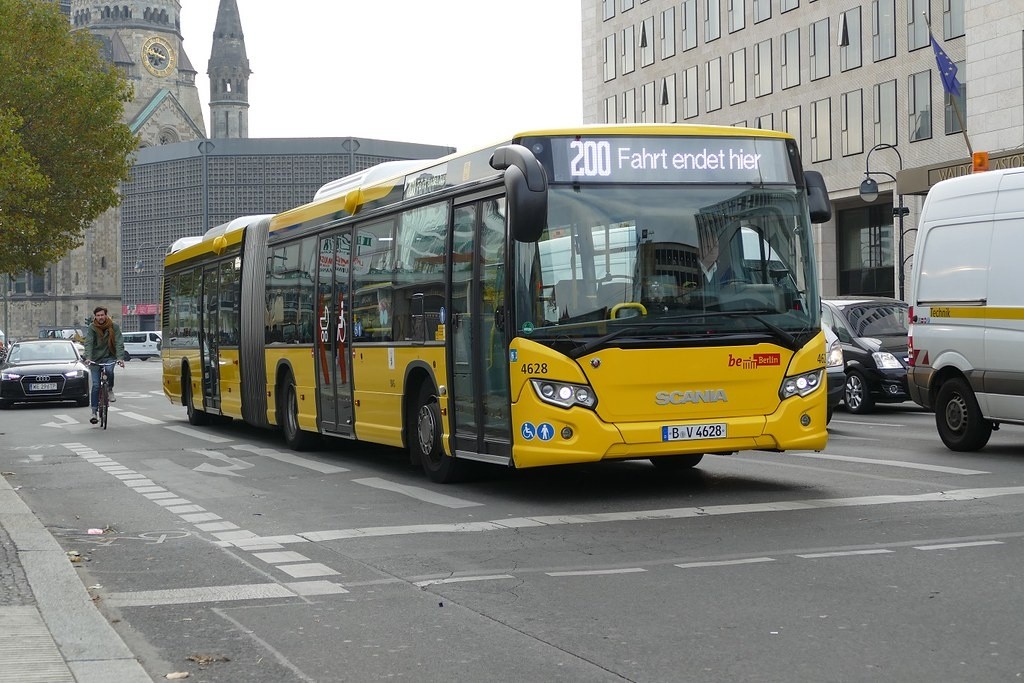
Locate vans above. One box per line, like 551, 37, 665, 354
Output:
121, 330, 161, 361
906, 166, 1024, 454
819, 295, 916, 427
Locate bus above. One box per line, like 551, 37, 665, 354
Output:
352, 281, 403, 342
159, 120, 833, 485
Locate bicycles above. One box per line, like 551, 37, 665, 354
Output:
86, 359, 122, 430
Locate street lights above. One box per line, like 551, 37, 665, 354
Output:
858, 143, 905, 329
134, 242, 160, 331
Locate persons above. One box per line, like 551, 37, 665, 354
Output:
48, 332, 56, 339
8, 341, 12, 349
355, 298, 393, 341
0, 339, 3, 351
676, 231, 740, 293
83, 306, 125, 424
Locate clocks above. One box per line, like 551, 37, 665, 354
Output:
141, 36, 177, 77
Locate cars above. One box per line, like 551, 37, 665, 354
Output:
0, 337, 90, 408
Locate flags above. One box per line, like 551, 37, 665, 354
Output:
931, 36, 961, 96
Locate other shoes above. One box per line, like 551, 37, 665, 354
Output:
90, 414, 98, 424
108, 390, 116, 402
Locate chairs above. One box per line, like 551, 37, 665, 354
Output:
595, 274, 633, 308
